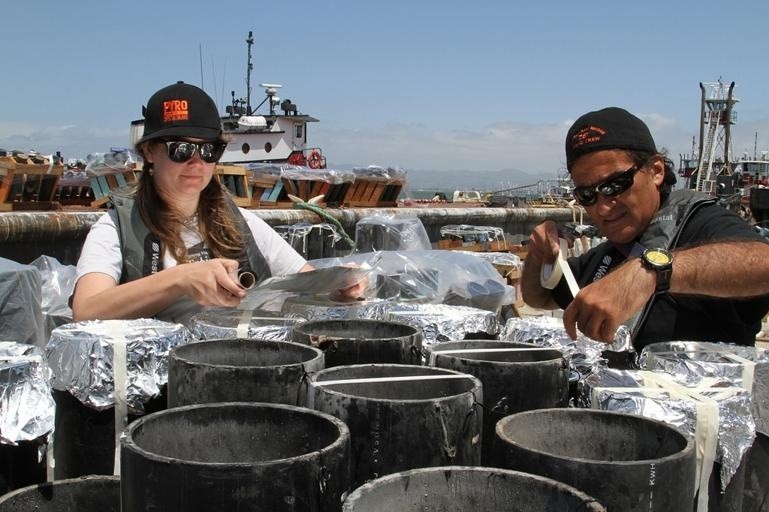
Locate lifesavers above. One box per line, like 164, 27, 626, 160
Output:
307, 151, 321, 169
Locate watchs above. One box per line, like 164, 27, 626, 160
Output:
639, 246, 675, 298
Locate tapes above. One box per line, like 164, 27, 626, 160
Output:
539, 238, 580, 298
237, 268, 259, 290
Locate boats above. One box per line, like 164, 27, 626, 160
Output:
127, 29, 327, 171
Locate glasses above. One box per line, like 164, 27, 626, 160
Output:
156, 136, 227, 163
573, 159, 647, 206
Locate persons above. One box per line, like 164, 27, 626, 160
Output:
71, 79, 372, 325
518, 104, 769, 354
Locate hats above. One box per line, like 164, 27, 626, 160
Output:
137, 80, 221, 144
566, 107, 656, 173
